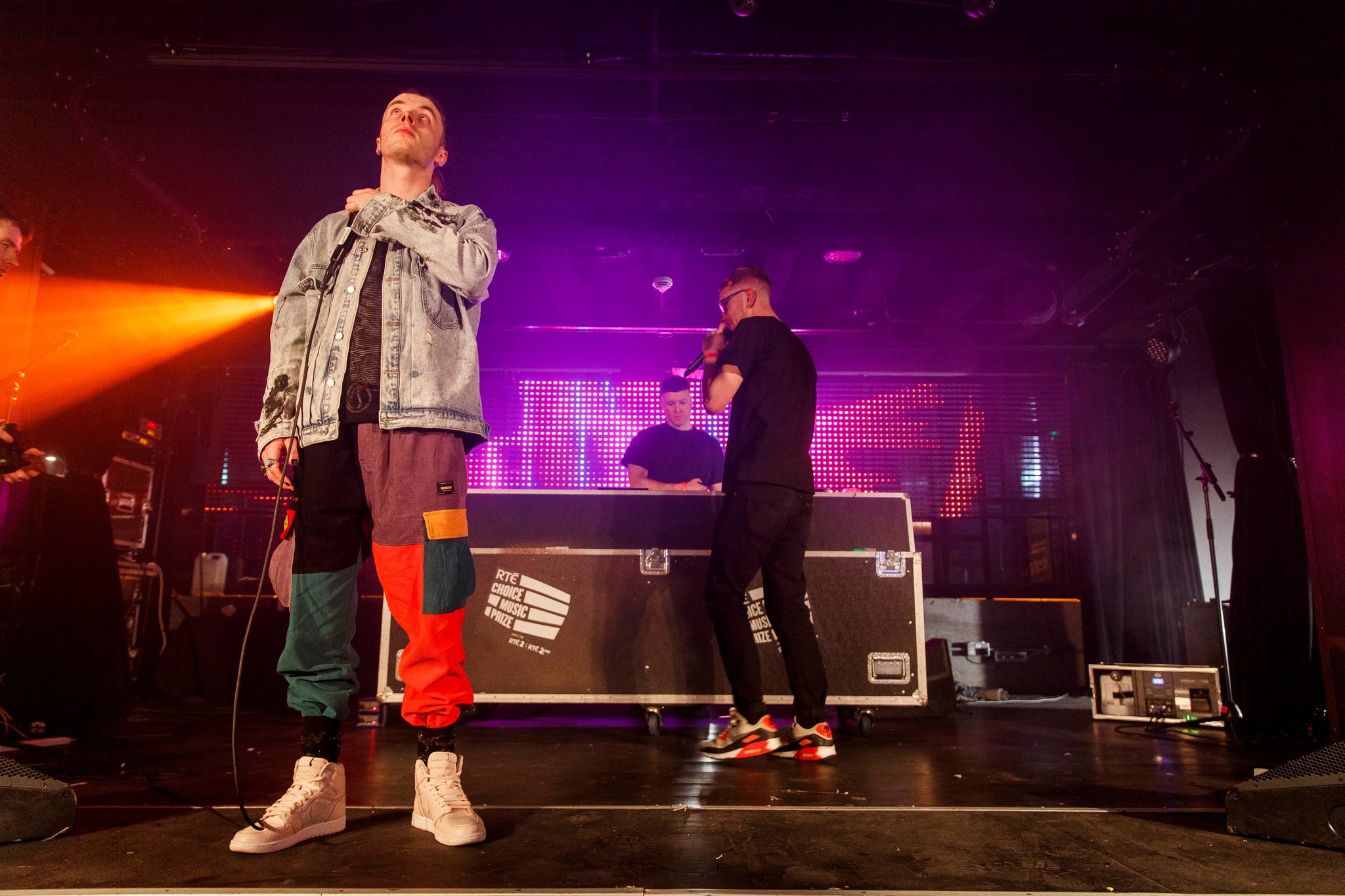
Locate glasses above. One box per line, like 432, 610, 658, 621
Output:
718, 290, 746, 314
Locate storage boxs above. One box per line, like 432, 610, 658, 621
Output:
100, 457, 155, 549
378, 488, 927, 735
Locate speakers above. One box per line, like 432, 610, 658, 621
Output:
0, 755, 76, 844
1224, 737, 1345, 849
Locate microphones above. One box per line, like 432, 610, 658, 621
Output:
323, 211, 361, 284
684, 326, 733, 378
1167, 390, 1176, 417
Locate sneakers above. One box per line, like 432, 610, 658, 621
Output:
696, 707, 786, 760
769, 717, 839, 761
410, 751, 488, 846
229, 758, 348, 853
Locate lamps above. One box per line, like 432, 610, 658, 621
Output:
964, 0, 995, 19
1148, 317, 1189, 365
728, 0, 759, 17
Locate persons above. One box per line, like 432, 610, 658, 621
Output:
0, 190, 46, 483
229, 86, 498, 853
694, 266, 837, 761
620, 375, 726, 490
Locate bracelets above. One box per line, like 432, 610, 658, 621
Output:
682, 482, 687, 490
704, 353, 719, 362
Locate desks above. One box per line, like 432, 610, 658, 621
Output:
167, 595, 384, 704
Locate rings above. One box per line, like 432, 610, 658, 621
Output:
263, 473, 266, 476
261, 465, 269, 471
265, 458, 279, 466
